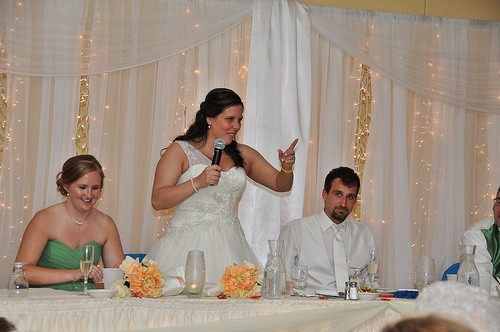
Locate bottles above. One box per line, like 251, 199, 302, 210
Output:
457, 245, 479, 287
262, 239, 287, 298
345, 281, 357, 300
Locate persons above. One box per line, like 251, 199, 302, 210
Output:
141, 88, 299, 298
460, 187, 500, 296
381, 315, 478, 332
265, 166, 380, 294
12, 155, 125, 292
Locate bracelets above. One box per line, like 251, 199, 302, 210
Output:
190, 177, 200, 193
280, 163, 294, 174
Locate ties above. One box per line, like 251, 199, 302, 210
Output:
331, 224, 349, 292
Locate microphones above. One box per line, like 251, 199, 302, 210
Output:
209, 138, 226, 186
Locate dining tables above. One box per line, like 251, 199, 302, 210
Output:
0, 287, 500, 332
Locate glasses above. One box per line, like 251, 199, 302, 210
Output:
493, 198, 500, 206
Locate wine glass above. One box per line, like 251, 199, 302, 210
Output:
367, 246, 380, 288
78, 244, 95, 296
7, 262, 30, 299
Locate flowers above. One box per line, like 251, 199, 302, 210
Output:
219, 259, 263, 299
112, 256, 164, 298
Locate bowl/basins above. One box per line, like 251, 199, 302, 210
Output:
86, 289, 116, 297
357, 288, 418, 301
496, 285, 500, 298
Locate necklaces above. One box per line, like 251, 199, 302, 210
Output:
64, 200, 92, 225
201, 143, 225, 156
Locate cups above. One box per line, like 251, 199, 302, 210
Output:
479, 274, 490, 295
124, 253, 146, 263
102, 268, 125, 289
416, 256, 434, 292
446, 273, 456, 280
184, 250, 205, 299
289, 265, 308, 296
